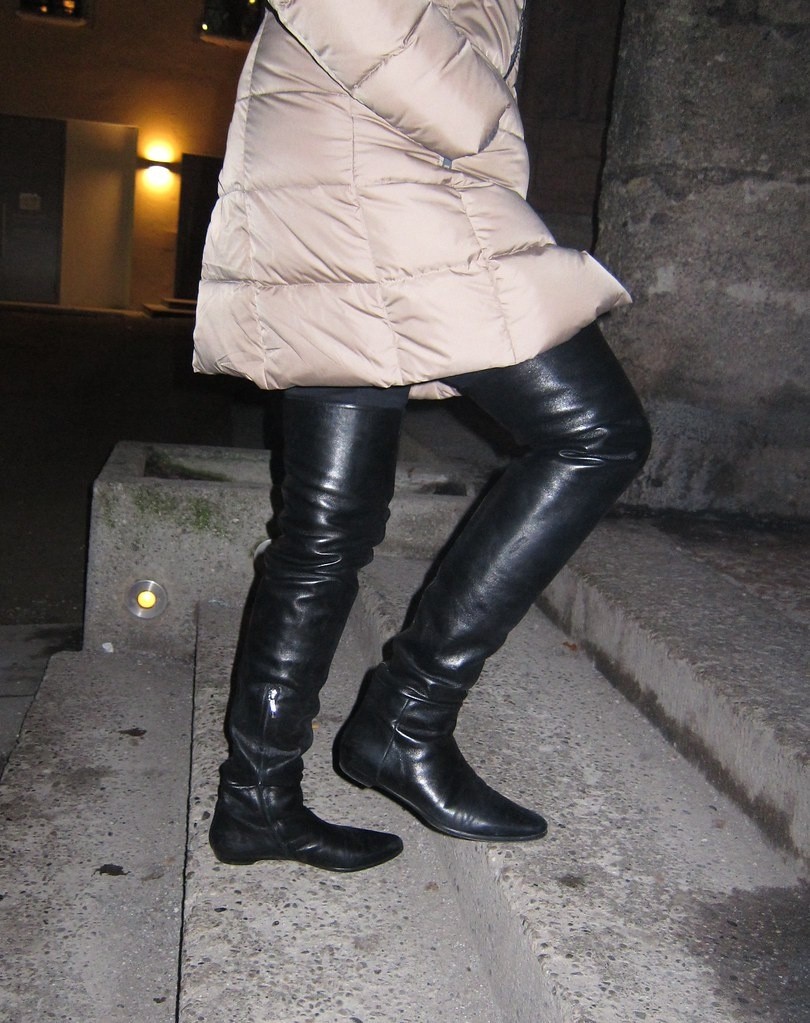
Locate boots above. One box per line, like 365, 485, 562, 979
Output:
209, 398, 405, 873
330, 317, 652, 845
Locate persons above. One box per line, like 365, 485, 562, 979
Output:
192, 1, 653, 873
